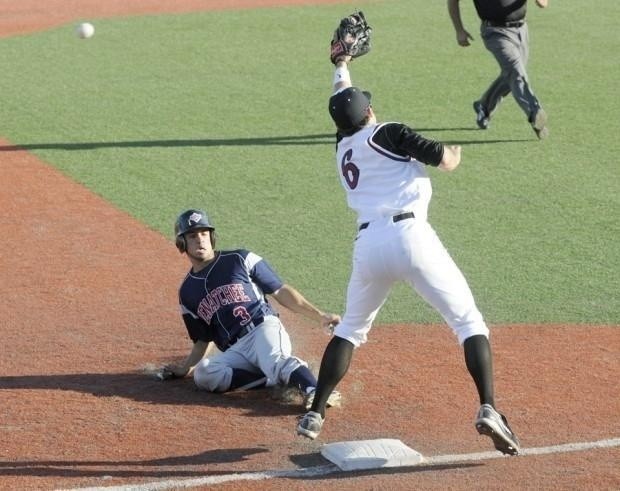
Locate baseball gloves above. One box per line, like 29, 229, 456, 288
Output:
331, 11, 372, 64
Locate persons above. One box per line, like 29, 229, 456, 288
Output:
446, 0, 551, 140
294, 9, 521, 454
140, 208, 346, 412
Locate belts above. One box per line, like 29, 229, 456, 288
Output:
222, 317, 264, 352
359, 212, 414, 230
484, 19, 524, 28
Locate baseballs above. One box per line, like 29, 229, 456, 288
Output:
78, 22, 95, 39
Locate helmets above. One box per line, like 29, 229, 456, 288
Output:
175, 209, 216, 253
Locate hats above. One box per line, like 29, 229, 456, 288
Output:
328, 87, 371, 132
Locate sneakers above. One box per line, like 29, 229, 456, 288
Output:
475, 404, 520, 457
298, 411, 327, 439
474, 101, 489, 129
304, 389, 341, 409
530, 106, 550, 140
